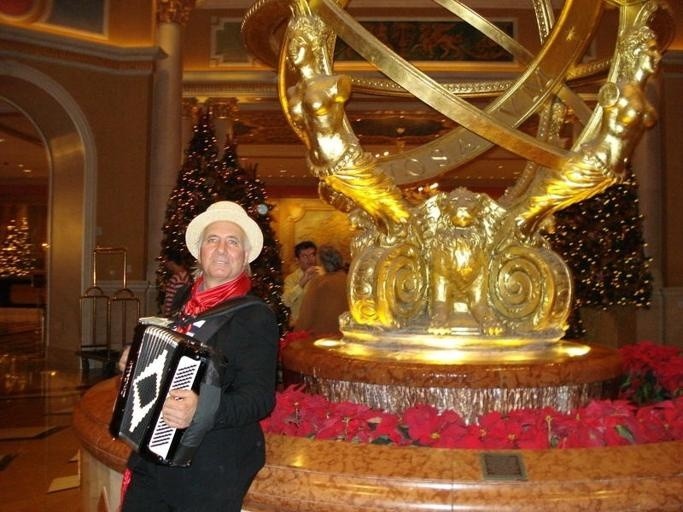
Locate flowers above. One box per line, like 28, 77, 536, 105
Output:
260, 340, 683, 450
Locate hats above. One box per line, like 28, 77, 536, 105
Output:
185, 201, 264, 264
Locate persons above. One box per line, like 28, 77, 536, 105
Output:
161, 251, 193, 318
281, 10, 414, 225
290, 245, 350, 337
280, 240, 327, 330
118, 200, 280, 512
520, 24, 661, 227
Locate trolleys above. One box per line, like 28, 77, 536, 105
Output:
73, 248, 142, 379
